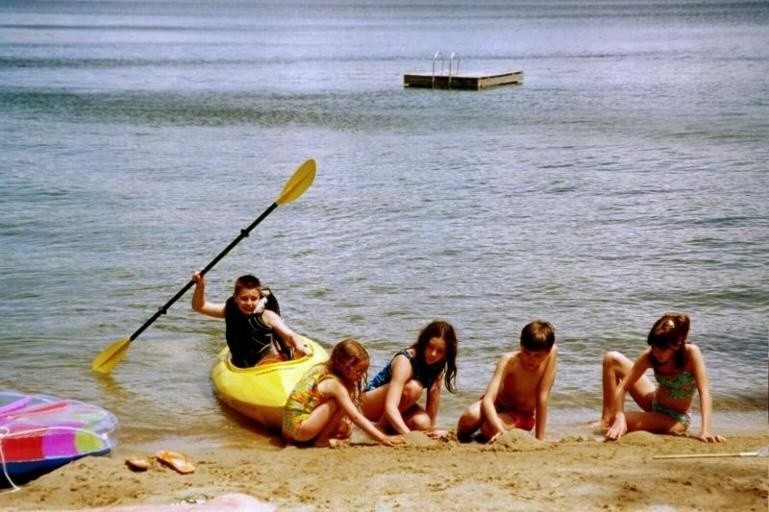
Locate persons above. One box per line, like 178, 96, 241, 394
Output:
281, 338, 404, 447
357, 321, 458, 436
455, 321, 558, 444
192, 271, 312, 368
590, 313, 728, 443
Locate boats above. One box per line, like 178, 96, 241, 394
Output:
213, 332, 331, 434
0, 388, 120, 477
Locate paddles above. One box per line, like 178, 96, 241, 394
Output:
93, 159, 316, 374
